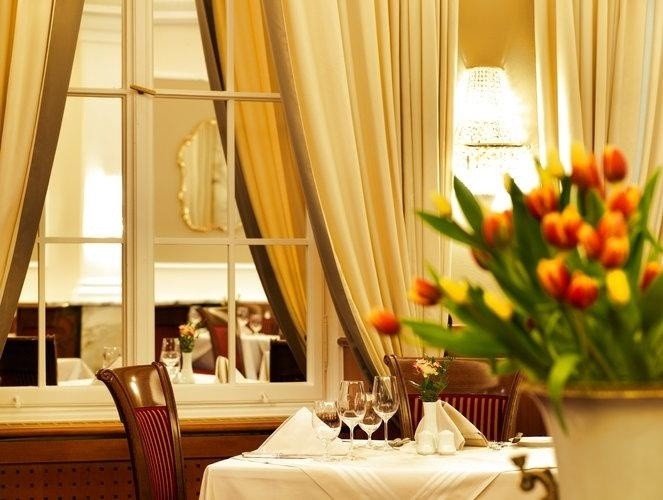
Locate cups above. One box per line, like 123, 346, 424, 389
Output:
415, 430, 436, 456
436, 430, 456, 456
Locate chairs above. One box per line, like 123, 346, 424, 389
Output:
262, 317, 276, 336
206, 320, 246, 379
0, 333, 58, 385
269, 339, 304, 381
383, 353, 525, 441
95, 362, 188, 500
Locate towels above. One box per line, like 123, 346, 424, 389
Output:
211, 356, 247, 384
241, 407, 348, 458
92, 356, 124, 384
414, 401, 488, 447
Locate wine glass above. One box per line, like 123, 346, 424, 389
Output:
355, 392, 383, 452
159, 337, 181, 384
372, 376, 400, 453
312, 400, 342, 464
336, 380, 367, 463
102, 346, 121, 367
236, 306, 262, 336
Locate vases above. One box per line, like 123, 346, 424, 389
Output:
414, 403, 439, 453
173, 352, 195, 384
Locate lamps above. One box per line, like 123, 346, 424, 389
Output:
447, 67, 541, 198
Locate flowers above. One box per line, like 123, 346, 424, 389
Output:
365, 140, 663, 441
408, 352, 453, 400
178, 319, 199, 352
516, 382, 663, 500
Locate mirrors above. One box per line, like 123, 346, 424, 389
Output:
173, 120, 245, 234
